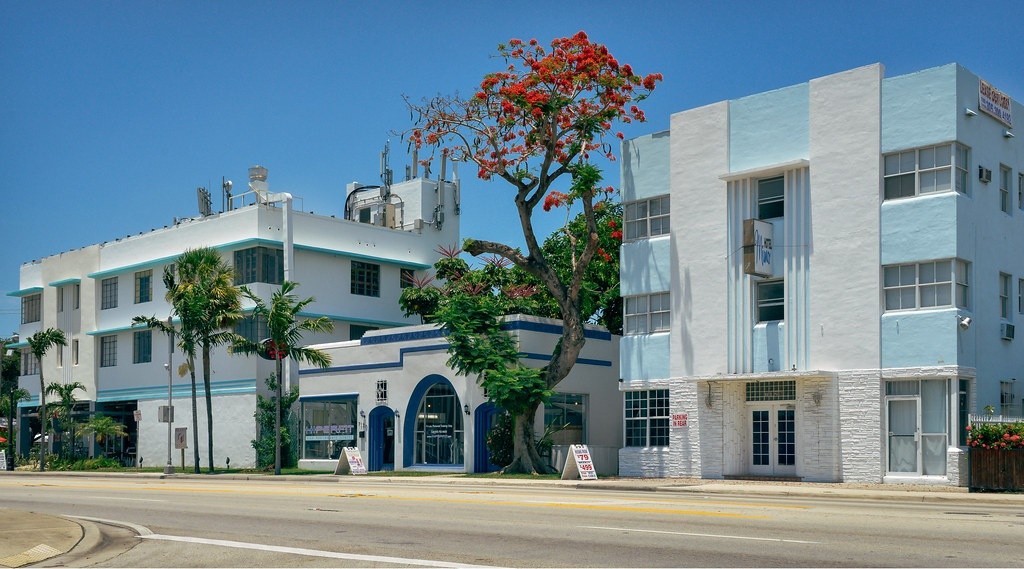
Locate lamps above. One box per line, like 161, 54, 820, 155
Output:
957, 314, 972, 329
360, 409, 366, 419
464, 404, 470, 415
395, 407, 400, 419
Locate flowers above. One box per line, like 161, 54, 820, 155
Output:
965, 405, 1024, 451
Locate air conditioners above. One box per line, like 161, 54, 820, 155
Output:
978, 167, 979, 182
1001, 323, 1015, 340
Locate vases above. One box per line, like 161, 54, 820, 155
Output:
970, 447, 1024, 494
